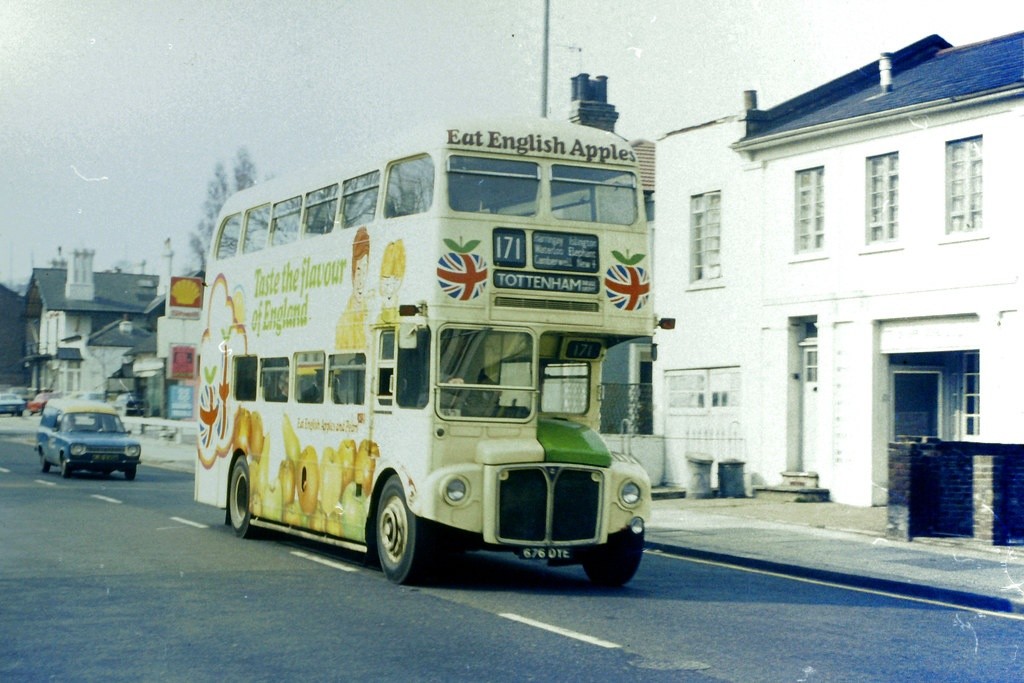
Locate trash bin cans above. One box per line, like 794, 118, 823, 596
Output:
684, 452, 714, 493
718, 461, 745, 498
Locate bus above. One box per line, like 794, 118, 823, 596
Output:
195, 114, 676, 591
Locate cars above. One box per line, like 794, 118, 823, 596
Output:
113, 393, 145, 417
0, 385, 56, 417
35, 397, 143, 480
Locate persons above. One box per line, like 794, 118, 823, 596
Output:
417, 329, 499, 416
272, 369, 301, 404
98, 417, 118, 433
